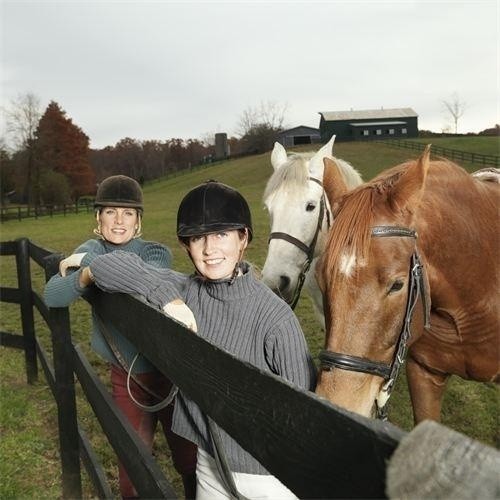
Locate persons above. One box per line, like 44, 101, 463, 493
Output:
89, 177, 319, 500
43, 175, 197, 500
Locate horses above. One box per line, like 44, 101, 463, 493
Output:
306, 142, 499, 429
256, 133, 362, 318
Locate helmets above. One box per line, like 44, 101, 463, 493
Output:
93, 174, 144, 215
176, 179, 254, 246
